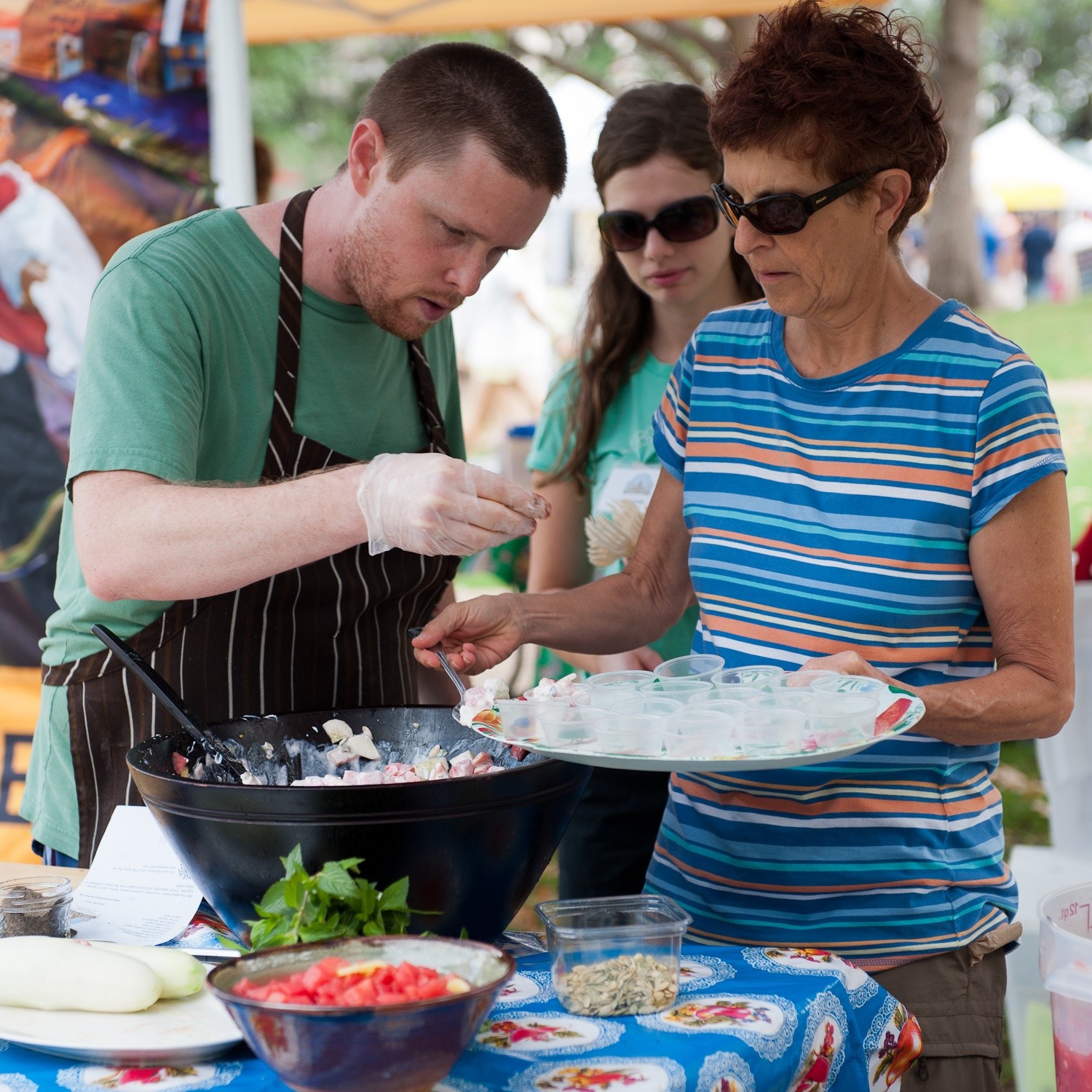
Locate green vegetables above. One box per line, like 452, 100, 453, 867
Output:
241, 842, 470, 955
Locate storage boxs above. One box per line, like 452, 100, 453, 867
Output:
533, 891, 695, 1019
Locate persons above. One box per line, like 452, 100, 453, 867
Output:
19, 42, 552, 871
406, 0, 1092, 1092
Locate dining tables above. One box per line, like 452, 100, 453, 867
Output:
1, 901, 926, 1092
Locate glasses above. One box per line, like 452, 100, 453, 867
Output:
597, 195, 722, 251
710, 178, 863, 235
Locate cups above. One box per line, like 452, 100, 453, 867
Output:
0, 875, 73, 938
495, 653, 890, 759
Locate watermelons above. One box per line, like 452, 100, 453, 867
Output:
227, 953, 455, 1009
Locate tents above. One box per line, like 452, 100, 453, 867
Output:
3, 0, 1089, 307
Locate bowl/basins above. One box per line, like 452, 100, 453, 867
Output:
533, 893, 693, 1017
207, 934, 516, 1091
127, 702, 598, 947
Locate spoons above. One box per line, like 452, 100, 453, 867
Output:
408, 625, 467, 722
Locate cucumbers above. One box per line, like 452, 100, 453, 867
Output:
0, 935, 211, 1012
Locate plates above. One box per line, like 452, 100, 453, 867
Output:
0, 947, 246, 1069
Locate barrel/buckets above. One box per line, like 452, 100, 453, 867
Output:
1038, 879, 1092, 1092
